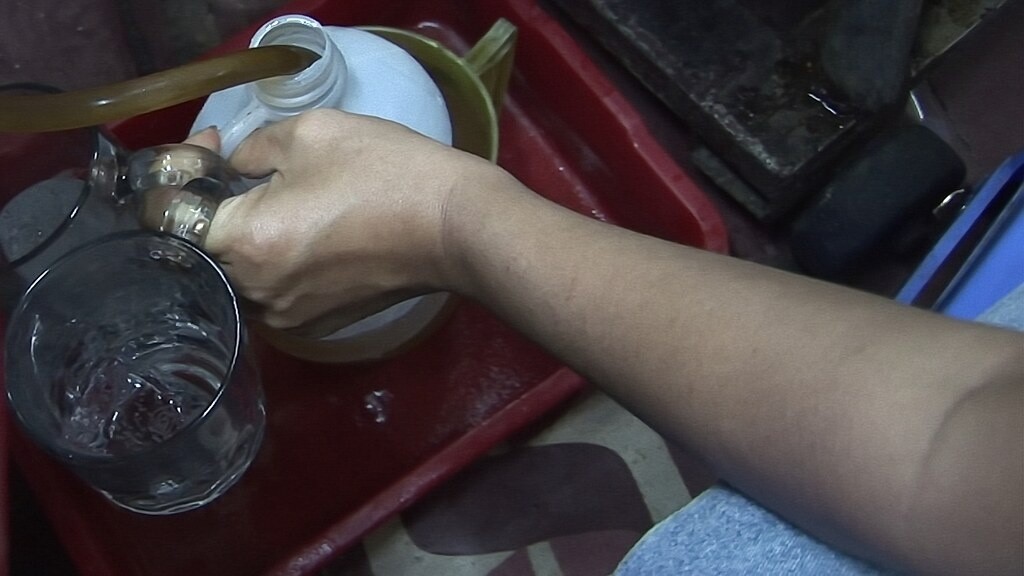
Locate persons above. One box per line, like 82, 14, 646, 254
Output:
141, 106, 1024, 576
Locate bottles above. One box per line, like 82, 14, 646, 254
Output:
187, 14, 453, 365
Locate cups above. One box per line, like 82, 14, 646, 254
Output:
0, 83, 249, 314
3, 185, 267, 518
256, 15, 517, 365
